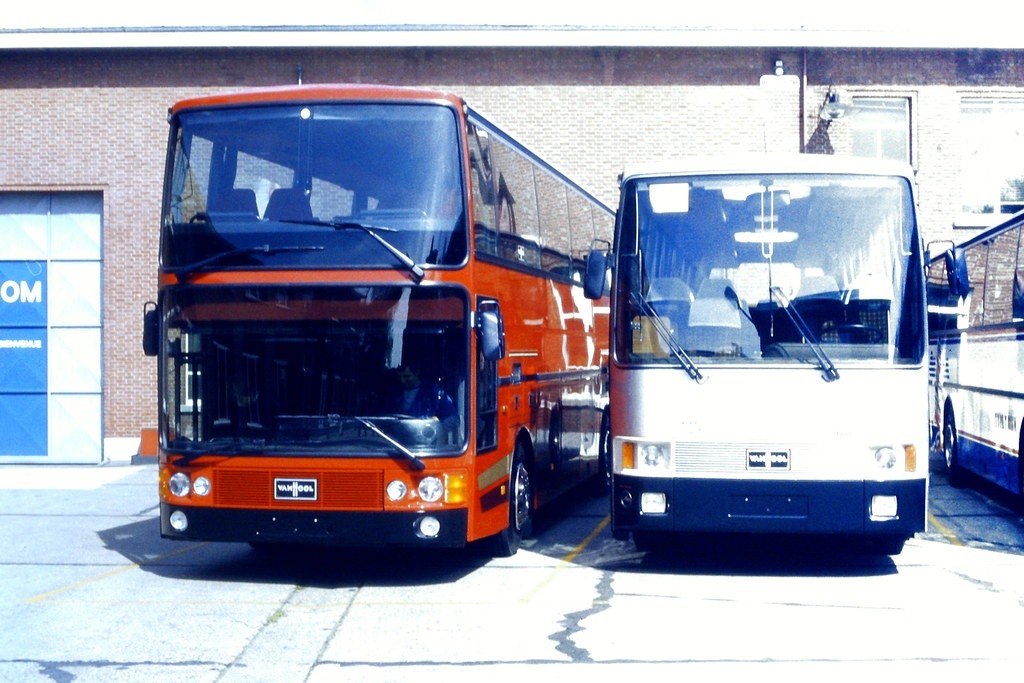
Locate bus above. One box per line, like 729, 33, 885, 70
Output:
922, 208, 1024, 522
608, 156, 928, 560
144, 86, 622, 558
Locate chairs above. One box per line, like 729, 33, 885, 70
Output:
207, 189, 433, 220
644, 275, 892, 360
